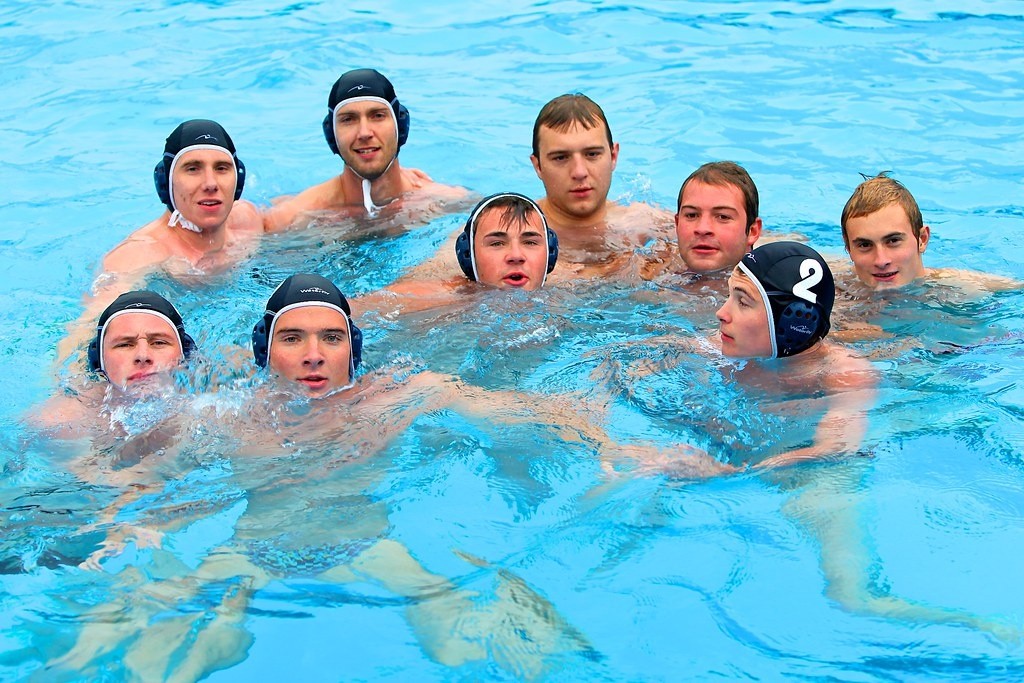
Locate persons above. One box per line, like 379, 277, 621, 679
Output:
87, 289, 197, 393
251, 273, 364, 397
674, 160, 765, 274
839, 176, 1023, 302
528, 92, 677, 288
453, 190, 560, 291
261, 67, 437, 234
662, 240, 873, 482
100, 119, 436, 280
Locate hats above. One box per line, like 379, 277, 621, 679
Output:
322, 69, 410, 155
153, 119, 245, 206
739, 240, 835, 361
249, 273, 362, 379
455, 192, 558, 290
86, 290, 197, 372
